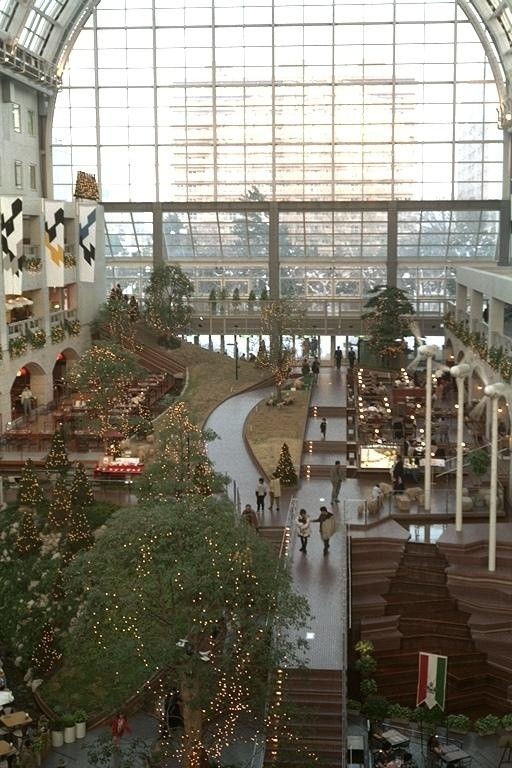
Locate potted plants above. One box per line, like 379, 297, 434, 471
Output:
390, 703, 512, 737
48, 708, 90, 748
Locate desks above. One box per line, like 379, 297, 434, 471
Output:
439, 744, 470, 767
381, 728, 410, 749
0, 711, 33, 756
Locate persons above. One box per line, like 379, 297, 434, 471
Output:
238, 352, 247, 361
241, 504, 258, 532
389, 453, 404, 494
268, 472, 282, 513
330, 460, 344, 503
312, 356, 320, 383
295, 507, 310, 552
110, 711, 133, 751
373, 484, 383, 501
348, 346, 357, 369
319, 416, 327, 438
254, 477, 268, 512
310, 504, 337, 556
333, 346, 343, 369
249, 352, 256, 362
301, 358, 310, 375
439, 417, 450, 441
